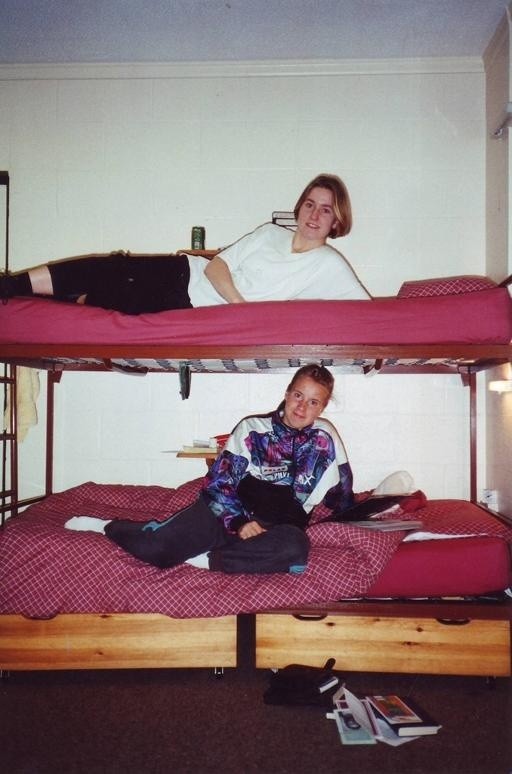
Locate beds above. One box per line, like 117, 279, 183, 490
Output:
1, 294, 510, 676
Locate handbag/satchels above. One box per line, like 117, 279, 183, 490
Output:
265, 658, 346, 709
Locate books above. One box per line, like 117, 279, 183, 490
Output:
365, 693, 443, 737
181, 445, 220, 454
271, 210, 298, 231
343, 519, 423, 533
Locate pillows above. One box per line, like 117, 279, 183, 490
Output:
394, 275, 502, 297
403, 490, 512, 542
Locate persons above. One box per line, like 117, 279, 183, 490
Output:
64, 364, 355, 573
0, 172, 375, 316
